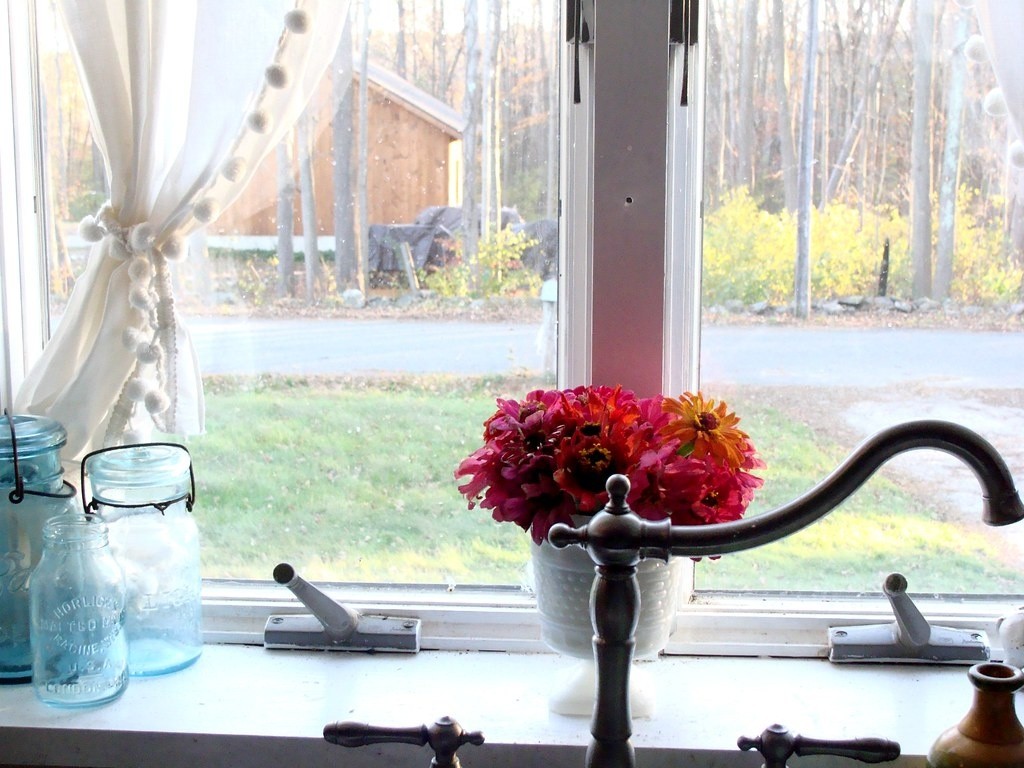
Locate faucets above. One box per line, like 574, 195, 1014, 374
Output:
547, 418, 1024, 565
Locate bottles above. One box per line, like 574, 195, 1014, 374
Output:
0, 405, 83, 684
27, 513, 130, 708
80, 441, 204, 678
924, 660, 1024, 768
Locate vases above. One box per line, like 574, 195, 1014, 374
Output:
529, 515, 684, 660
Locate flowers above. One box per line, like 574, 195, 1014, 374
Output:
454, 385, 767, 562
